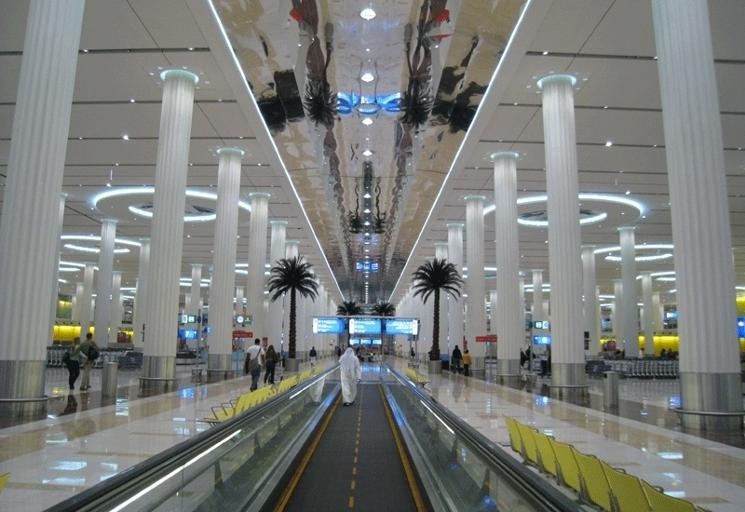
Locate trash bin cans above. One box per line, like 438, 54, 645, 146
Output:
602, 371, 621, 409
101, 362, 118, 397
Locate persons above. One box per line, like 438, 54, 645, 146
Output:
638, 345, 646, 361
399, 344, 404, 358
667, 347, 675, 359
261, 344, 278, 386
411, 347, 416, 359
341, 343, 346, 355
68, 333, 101, 389
660, 347, 666, 357
520, 346, 526, 368
243, 338, 266, 391
64, 335, 88, 390
452, 344, 463, 374
526, 345, 537, 371
614, 345, 622, 355
602, 346, 613, 360
309, 345, 318, 368
463, 348, 473, 376
335, 343, 342, 361
337, 346, 362, 406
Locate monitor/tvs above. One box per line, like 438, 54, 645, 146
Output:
187, 315, 196, 323
534, 320, 543, 329
236, 315, 244, 323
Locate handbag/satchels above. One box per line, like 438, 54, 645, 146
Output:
88, 346, 99, 359
63, 352, 70, 363
249, 358, 258, 371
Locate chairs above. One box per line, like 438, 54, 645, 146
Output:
197, 362, 328, 429
501, 410, 712, 511
46, 345, 143, 371
524, 353, 678, 380
403, 363, 431, 391
